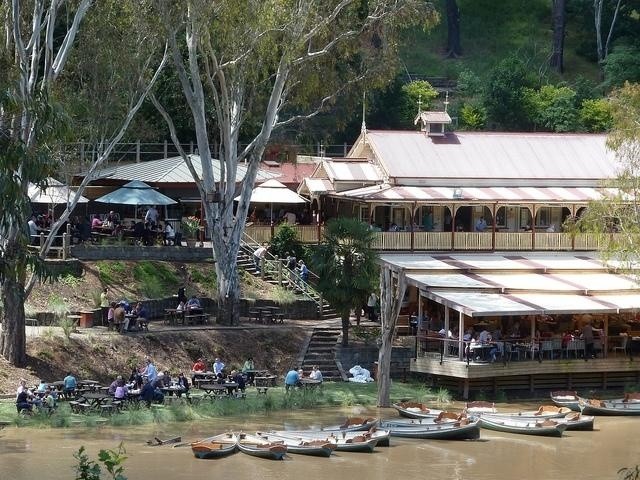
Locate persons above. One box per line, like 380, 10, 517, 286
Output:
310, 364, 324, 385
191, 358, 206, 387
241, 357, 255, 384
107, 359, 190, 408
423, 309, 431, 328
212, 357, 225, 379
15, 370, 77, 416
252, 245, 271, 274
284, 366, 299, 385
247, 202, 312, 225
422, 210, 437, 231
298, 369, 304, 379
367, 220, 398, 233
367, 293, 379, 322
546, 223, 556, 232
474, 215, 489, 232
176, 297, 186, 312
24, 206, 176, 246
100, 287, 150, 331
408, 311, 418, 328
282, 250, 309, 294
227, 369, 246, 395
438, 319, 604, 363
186, 295, 201, 308
444, 213, 452, 231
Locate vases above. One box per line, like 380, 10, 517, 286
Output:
186, 239, 197, 249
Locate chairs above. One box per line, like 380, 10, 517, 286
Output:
504, 334, 628, 362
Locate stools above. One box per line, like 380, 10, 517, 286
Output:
109, 305, 288, 332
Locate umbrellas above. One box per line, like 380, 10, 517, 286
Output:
233, 179, 313, 221
24, 176, 91, 217
94, 179, 179, 222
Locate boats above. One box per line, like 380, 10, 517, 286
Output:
193, 429, 237, 459
268, 390, 639, 454
255, 430, 337, 457
235, 428, 289, 460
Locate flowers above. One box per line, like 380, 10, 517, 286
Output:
181, 215, 204, 238
